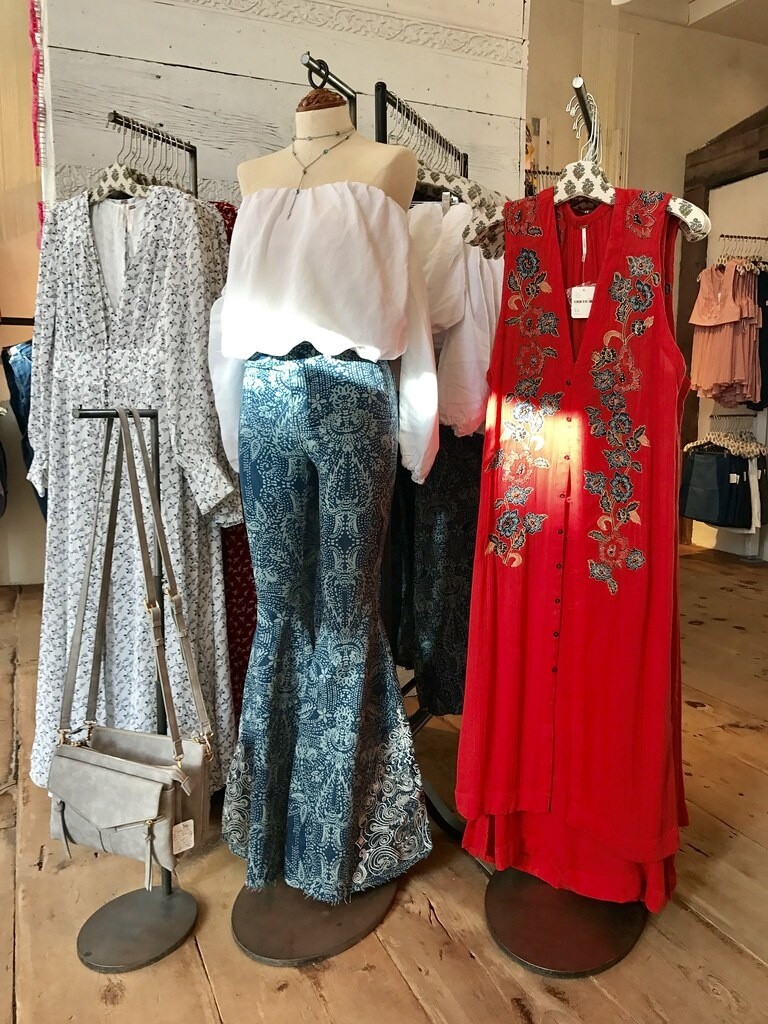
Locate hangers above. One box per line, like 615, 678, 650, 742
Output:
385, 92, 768, 276
683, 414, 768, 458
59, 113, 219, 223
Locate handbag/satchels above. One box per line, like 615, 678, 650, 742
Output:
46, 741, 197, 893
86, 723, 215, 850
